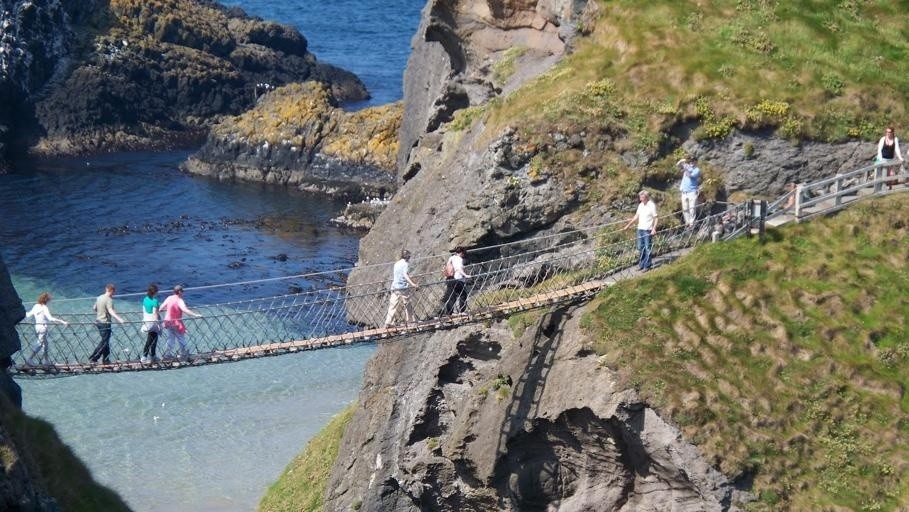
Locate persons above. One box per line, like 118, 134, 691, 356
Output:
624, 190, 658, 273
88, 284, 125, 366
140, 286, 163, 363
446, 247, 470, 315
878, 127, 902, 189
25, 292, 68, 366
385, 250, 420, 329
675, 157, 701, 231
159, 285, 202, 360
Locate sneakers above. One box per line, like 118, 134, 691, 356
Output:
384, 323, 399, 330
684, 225, 699, 233
635, 266, 652, 273
25, 358, 56, 369
140, 352, 195, 364
88, 360, 118, 366
449, 311, 469, 318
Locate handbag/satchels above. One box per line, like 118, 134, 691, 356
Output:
443, 255, 455, 279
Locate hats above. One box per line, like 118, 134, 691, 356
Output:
686, 156, 699, 162
